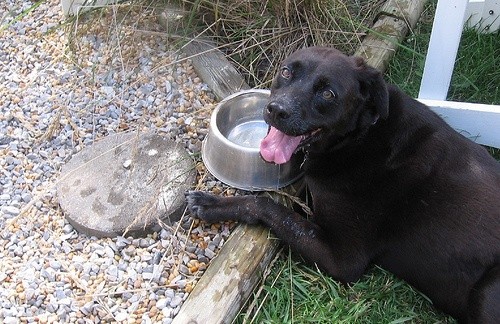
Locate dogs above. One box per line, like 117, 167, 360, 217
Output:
183, 45, 500, 324
